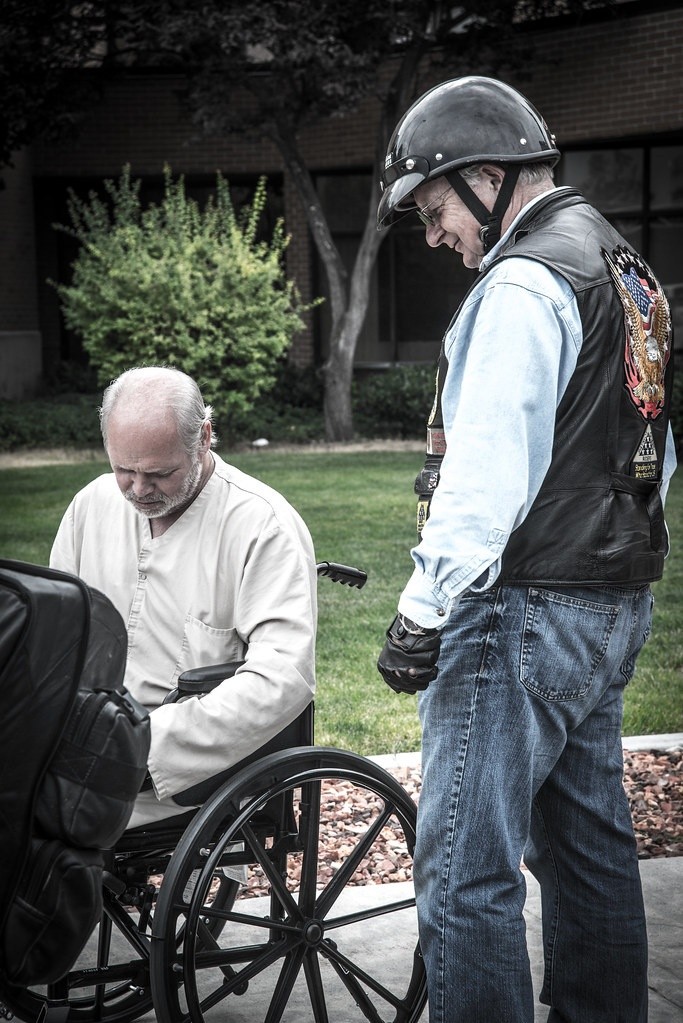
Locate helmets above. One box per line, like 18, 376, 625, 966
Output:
377, 74, 560, 232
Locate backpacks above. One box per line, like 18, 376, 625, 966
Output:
0, 558, 152, 990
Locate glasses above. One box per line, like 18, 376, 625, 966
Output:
417, 163, 478, 227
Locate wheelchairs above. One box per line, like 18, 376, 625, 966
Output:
0, 561, 428, 1023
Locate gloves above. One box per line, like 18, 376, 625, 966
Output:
376, 615, 443, 695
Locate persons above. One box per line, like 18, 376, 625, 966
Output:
374, 71, 669, 1023
44, 366, 318, 837
